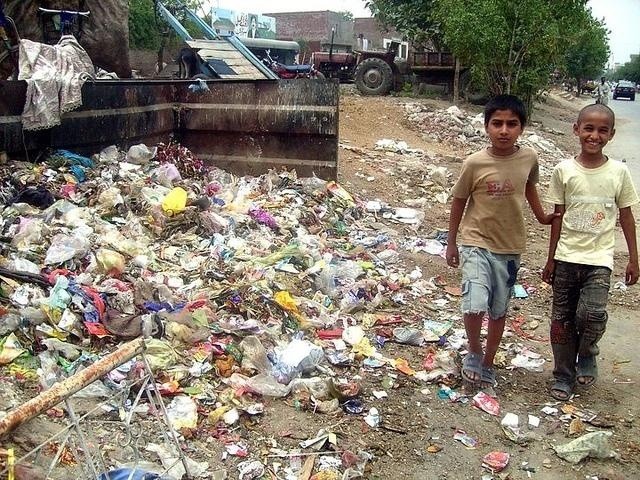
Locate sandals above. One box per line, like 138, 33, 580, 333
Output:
461, 351, 484, 383
550, 379, 577, 402
575, 354, 599, 388
473, 366, 497, 389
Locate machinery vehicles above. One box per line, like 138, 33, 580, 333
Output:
311, 24, 528, 103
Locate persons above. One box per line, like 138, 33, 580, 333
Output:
247, 15, 260, 38
592, 76, 609, 105
567, 76, 574, 91
540, 102, 640, 401
445, 93, 561, 389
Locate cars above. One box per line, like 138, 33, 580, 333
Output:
613, 82, 635, 100
